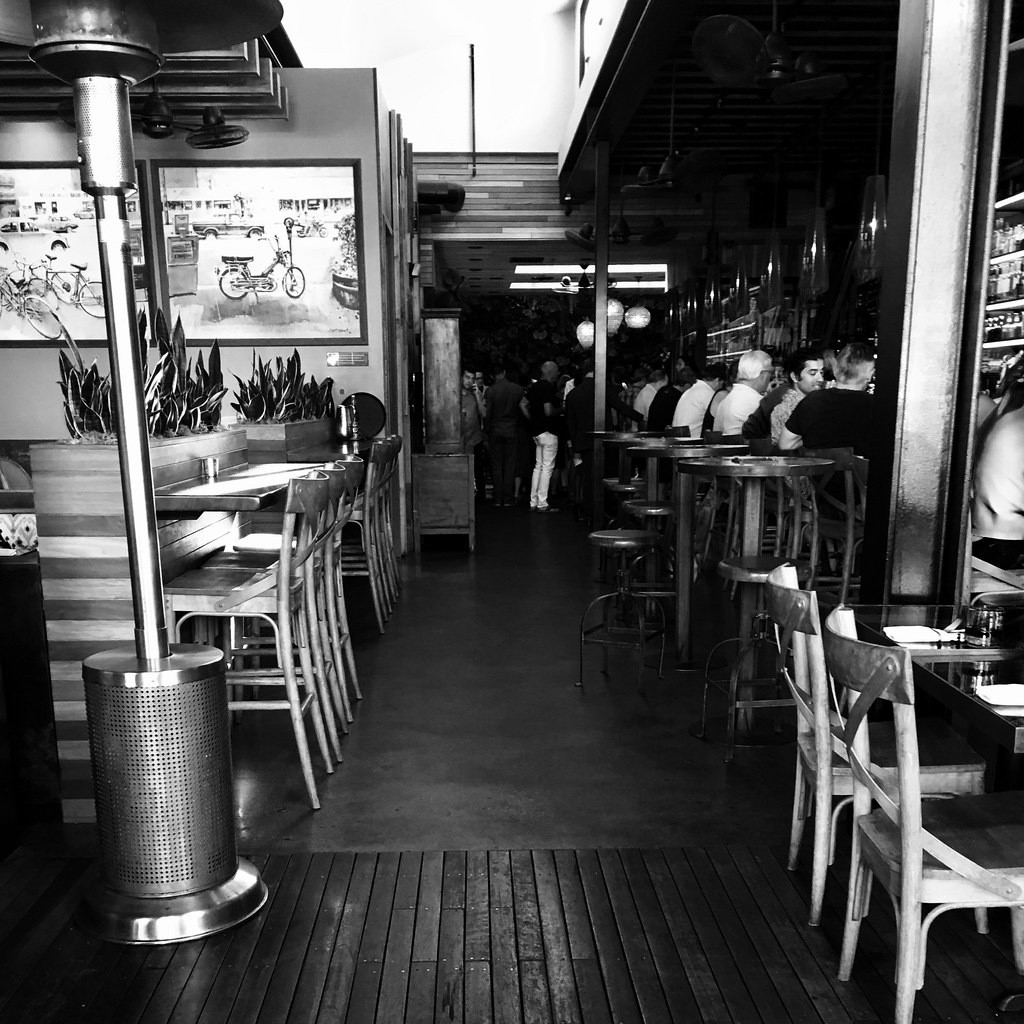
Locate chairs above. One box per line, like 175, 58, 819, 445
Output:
767, 561, 986, 926
163, 434, 404, 810
821, 603, 1024, 1024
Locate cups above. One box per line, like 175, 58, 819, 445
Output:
201, 459, 219, 480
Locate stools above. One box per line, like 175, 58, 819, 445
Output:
578, 477, 864, 690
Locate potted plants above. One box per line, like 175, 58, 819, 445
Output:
27, 305, 335, 714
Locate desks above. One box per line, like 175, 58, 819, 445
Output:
154, 461, 321, 522
839, 602, 1024, 755
590, 430, 837, 675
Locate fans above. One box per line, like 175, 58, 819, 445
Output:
566, 0, 853, 256
132, 106, 249, 149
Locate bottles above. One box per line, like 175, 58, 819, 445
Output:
982, 312, 1024, 342
351, 395, 361, 441
986, 218, 1024, 304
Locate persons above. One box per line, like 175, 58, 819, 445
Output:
458, 345, 876, 599
969, 369, 1024, 649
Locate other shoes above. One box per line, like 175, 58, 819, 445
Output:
663, 482, 730, 501
528, 508, 536, 511
493, 504, 501, 506
503, 497, 518, 506
537, 506, 559, 512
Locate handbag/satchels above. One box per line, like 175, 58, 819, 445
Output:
700, 387, 727, 437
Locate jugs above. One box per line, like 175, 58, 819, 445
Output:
335, 404, 354, 440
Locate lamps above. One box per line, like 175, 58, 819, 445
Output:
140, 73, 173, 138
